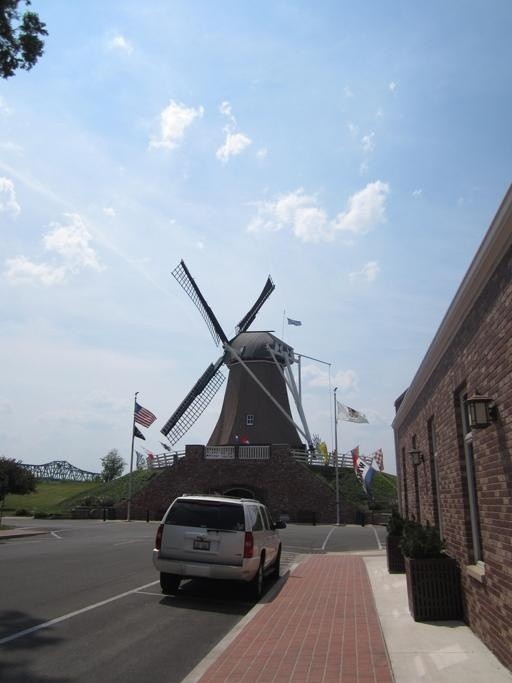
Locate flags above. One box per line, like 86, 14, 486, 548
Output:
286, 316, 302, 326
136, 451, 143, 465
336, 399, 369, 424
360, 455, 373, 466
161, 442, 171, 451
143, 447, 154, 462
232, 432, 250, 446
317, 441, 328, 463
350, 446, 358, 464
373, 447, 384, 472
133, 425, 145, 439
134, 401, 157, 428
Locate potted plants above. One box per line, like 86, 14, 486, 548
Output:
385, 505, 463, 622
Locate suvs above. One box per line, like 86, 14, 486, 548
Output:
152, 493, 286, 595
